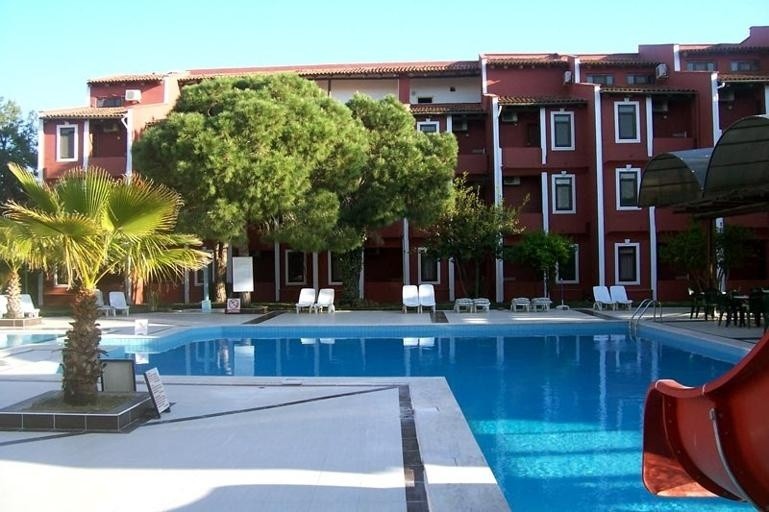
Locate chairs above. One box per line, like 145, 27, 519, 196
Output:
109, 292, 130, 317
453, 295, 552, 313
295, 288, 317, 314
93, 289, 113, 318
687, 286, 769, 328
402, 285, 420, 313
21, 294, 40, 318
593, 285, 616, 311
0, 295, 11, 316
610, 285, 634, 310
314, 288, 335, 315
419, 284, 436, 313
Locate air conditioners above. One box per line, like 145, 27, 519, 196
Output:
720, 89, 735, 102
502, 112, 518, 123
504, 175, 522, 185
104, 121, 120, 133
562, 71, 572, 85
125, 89, 141, 101
453, 121, 469, 132
653, 102, 668, 112
655, 64, 667, 79
